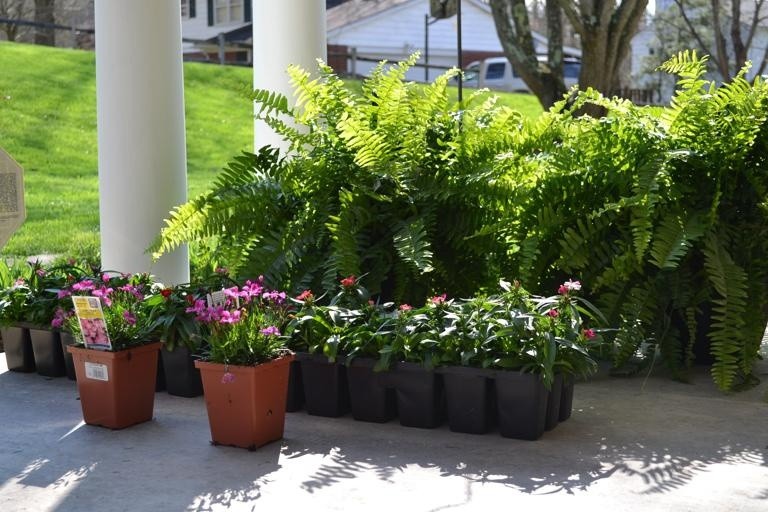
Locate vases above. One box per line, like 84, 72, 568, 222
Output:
194, 353, 295, 451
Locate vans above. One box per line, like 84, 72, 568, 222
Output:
447, 55, 591, 97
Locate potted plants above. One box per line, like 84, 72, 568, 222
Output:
629, 50, 768, 392
